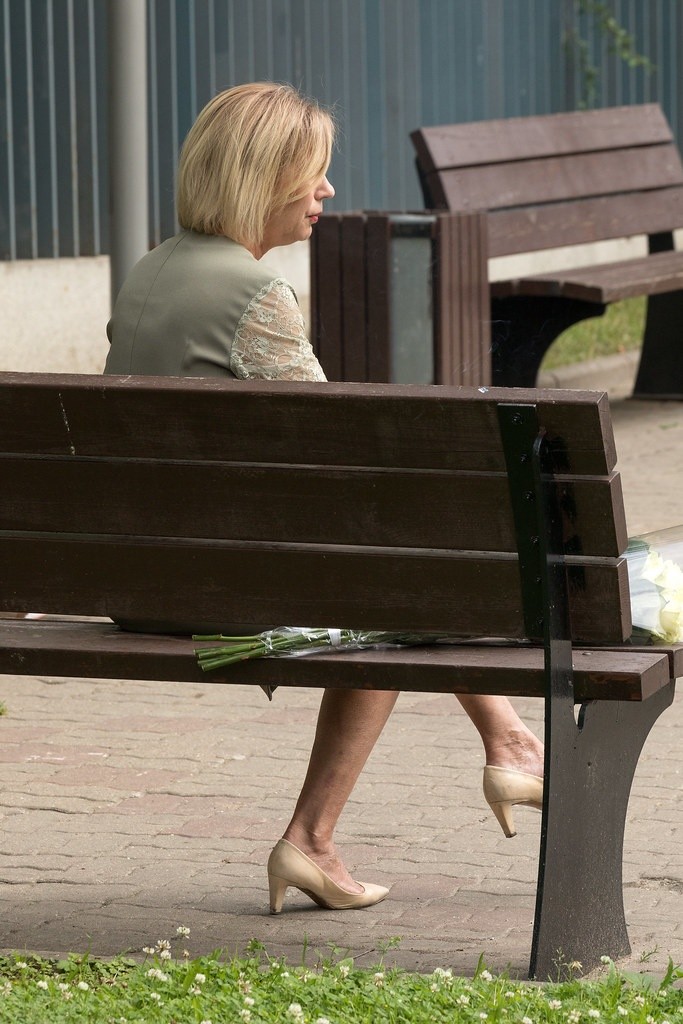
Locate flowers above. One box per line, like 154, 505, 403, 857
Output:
192, 538, 683, 672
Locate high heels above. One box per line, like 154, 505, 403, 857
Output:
484, 766, 545, 838
267, 838, 391, 913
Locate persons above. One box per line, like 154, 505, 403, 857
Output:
103, 82, 544, 913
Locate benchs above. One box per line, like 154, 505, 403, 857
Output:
406, 102, 683, 403
0, 371, 683, 979
